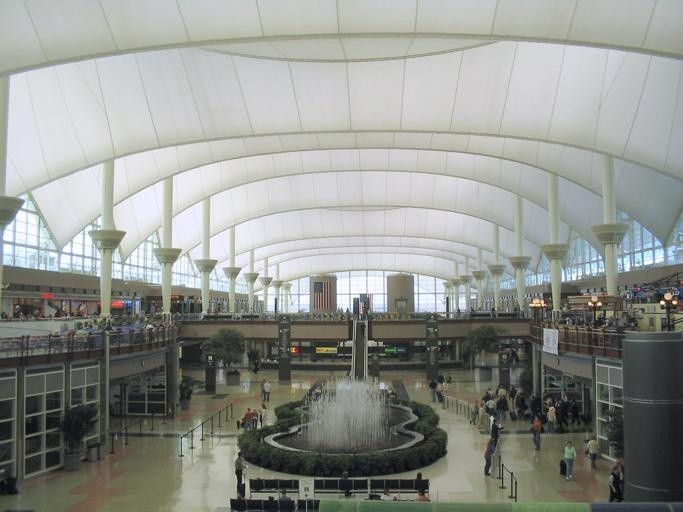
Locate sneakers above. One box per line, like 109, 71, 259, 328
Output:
565, 475, 572, 479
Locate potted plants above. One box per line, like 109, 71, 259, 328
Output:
56, 399, 99, 471
474, 366, 492, 383
227, 370, 241, 386
178, 381, 192, 410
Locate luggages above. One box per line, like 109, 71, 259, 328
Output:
560, 460, 566, 475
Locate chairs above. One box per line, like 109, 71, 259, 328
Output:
230, 478, 429, 512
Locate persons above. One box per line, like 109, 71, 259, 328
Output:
567, 316, 675, 333
158, 382, 193, 409
235, 451, 430, 502
242, 380, 271, 430
1, 305, 165, 348
429, 348, 624, 503
